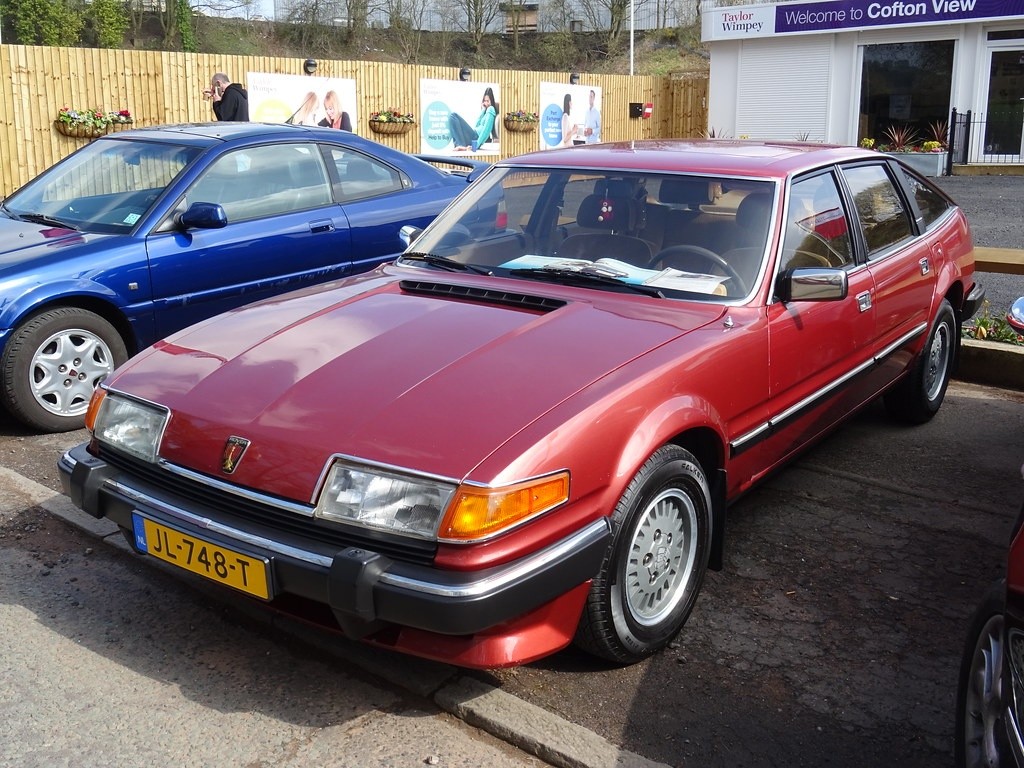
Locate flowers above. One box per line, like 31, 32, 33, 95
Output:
59, 108, 133, 129
861, 137, 946, 153
506, 111, 540, 121
371, 110, 414, 123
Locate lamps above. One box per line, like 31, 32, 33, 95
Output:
304, 60, 317, 73
571, 74, 580, 84
460, 68, 471, 80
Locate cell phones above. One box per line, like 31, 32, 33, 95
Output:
214, 86, 222, 96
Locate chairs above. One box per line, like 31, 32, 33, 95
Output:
258, 157, 317, 211
710, 191, 833, 280
185, 159, 239, 209
252, 152, 379, 210
557, 193, 653, 267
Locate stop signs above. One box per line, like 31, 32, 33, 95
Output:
642, 101, 654, 119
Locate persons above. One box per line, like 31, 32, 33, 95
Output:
295, 89, 352, 132
447, 87, 498, 151
562, 93, 578, 148
211, 72, 248, 121
583, 89, 601, 145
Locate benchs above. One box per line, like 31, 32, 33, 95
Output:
647, 177, 848, 260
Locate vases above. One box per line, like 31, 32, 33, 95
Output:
54, 120, 132, 139
369, 121, 413, 134
888, 151, 950, 177
504, 120, 537, 132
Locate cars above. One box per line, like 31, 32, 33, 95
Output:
55, 137, 986, 671
0, 123, 508, 431
